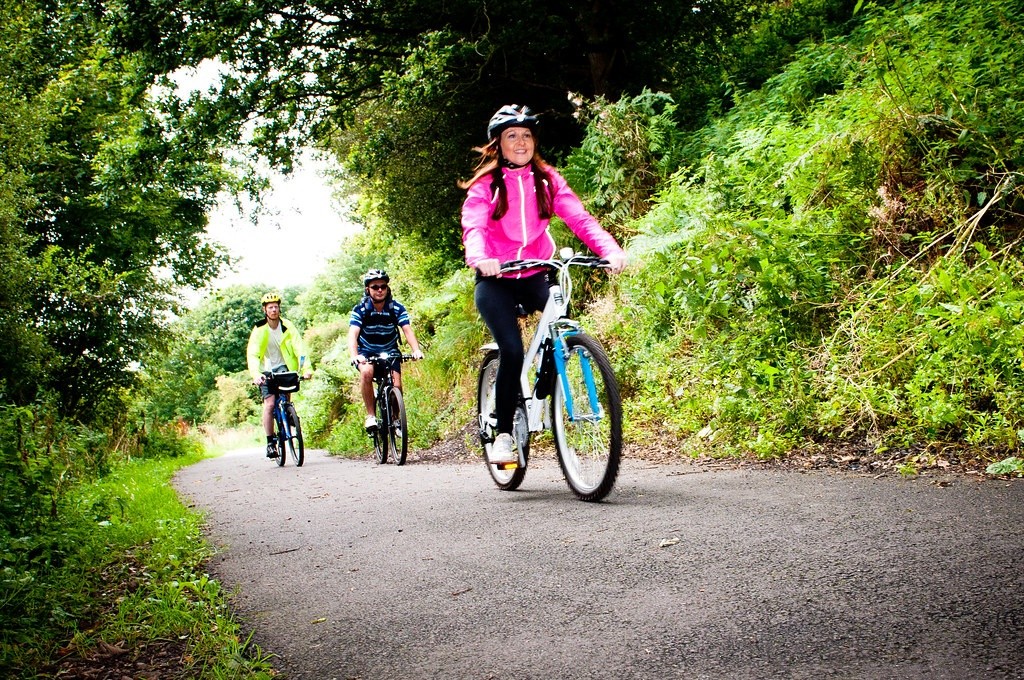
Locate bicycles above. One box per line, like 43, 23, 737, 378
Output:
252, 371, 304, 467
351, 355, 422, 465
476, 247, 627, 502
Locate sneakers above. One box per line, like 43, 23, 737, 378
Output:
490, 432, 514, 464
266, 445, 278, 457
392, 419, 402, 437
364, 414, 378, 429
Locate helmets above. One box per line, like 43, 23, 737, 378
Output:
487, 103, 543, 142
260, 293, 281, 306
364, 269, 389, 286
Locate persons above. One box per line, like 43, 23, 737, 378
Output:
246, 293, 315, 459
346, 269, 426, 438
459, 103, 627, 465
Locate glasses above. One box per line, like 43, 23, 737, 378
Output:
368, 284, 387, 289
